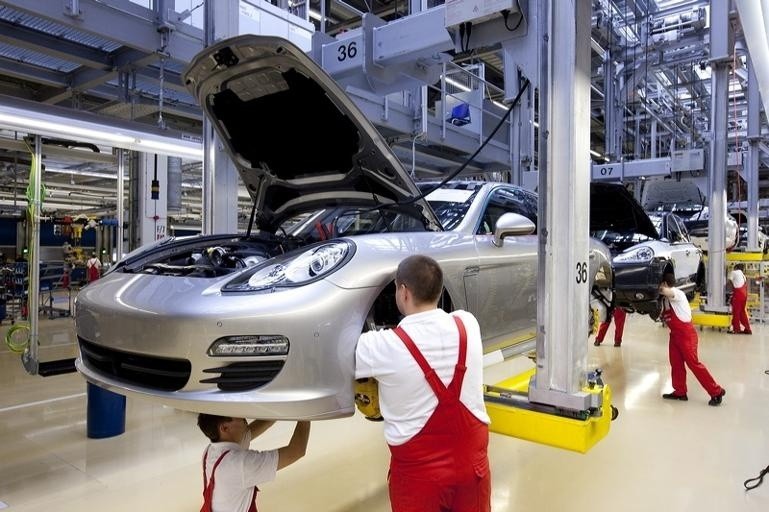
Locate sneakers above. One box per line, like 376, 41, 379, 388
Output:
663, 393, 688, 400
709, 390, 725, 405
595, 339, 620, 346
727, 330, 751, 334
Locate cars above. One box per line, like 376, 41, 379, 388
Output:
71, 30, 614, 423
590, 180, 768, 318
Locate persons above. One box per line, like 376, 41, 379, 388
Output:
87, 254, 102, 283
592, 306, 628, 348
350, 254, 493, 512
657, 270, 727, 408
195, 413, 313, 511
724, 263, 753, 335
